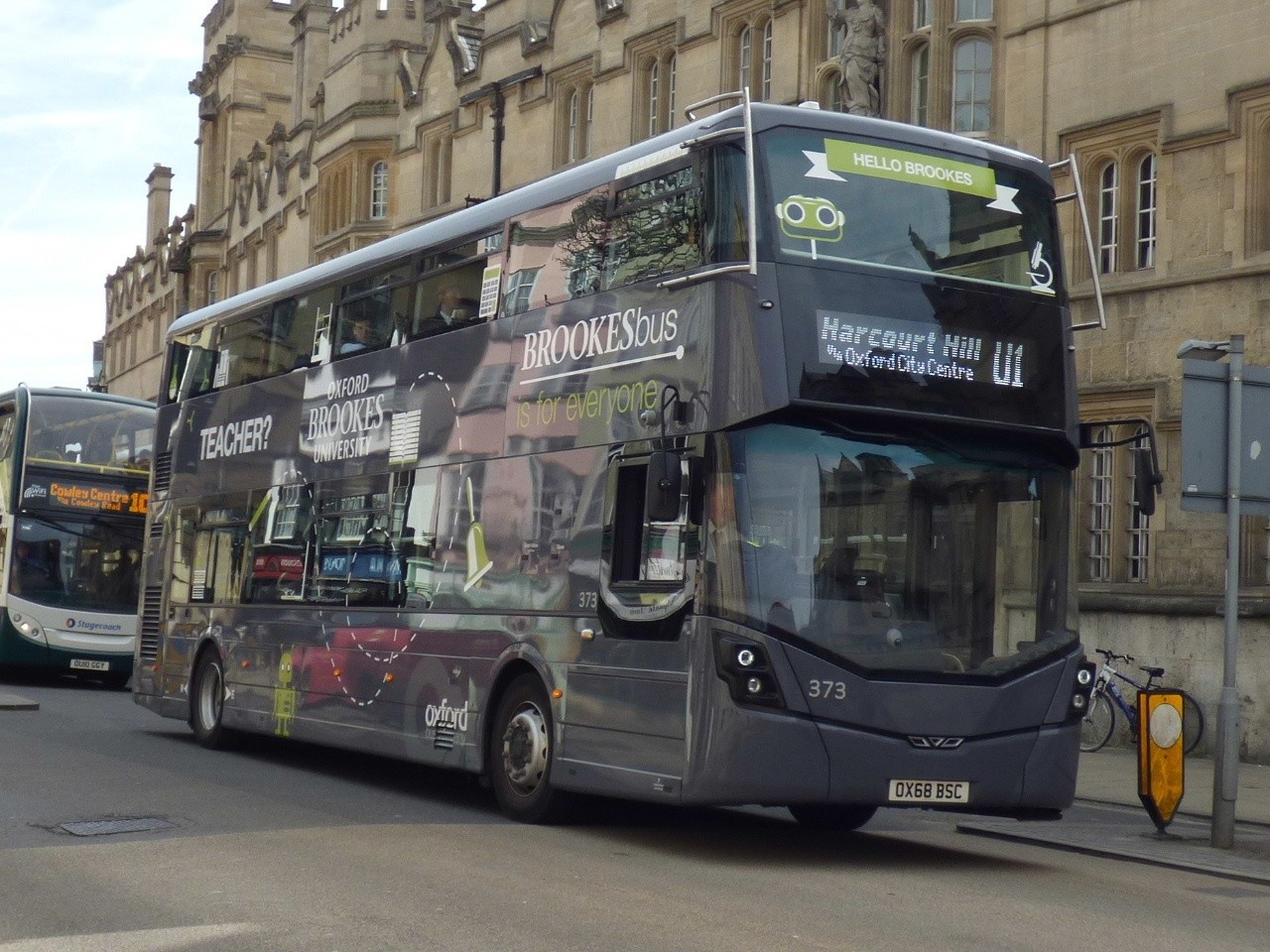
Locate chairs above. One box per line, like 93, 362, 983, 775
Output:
34, 450, 149, 472
240, 355, 313, 383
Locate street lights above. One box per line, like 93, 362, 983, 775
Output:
1175, 335, 1246, 849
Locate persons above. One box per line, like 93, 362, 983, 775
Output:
342, 276, 480, 357
11, 430, 150, 603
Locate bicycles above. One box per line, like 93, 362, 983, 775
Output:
1080, 648, 1204, 756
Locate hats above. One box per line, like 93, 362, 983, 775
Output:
435, 277, 470, 294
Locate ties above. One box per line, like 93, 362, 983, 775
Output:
715, 531, 729, 563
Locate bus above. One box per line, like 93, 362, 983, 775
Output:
0, 383, 165, 687
242, 472, 416, 607
131, 86, 1166, 827
